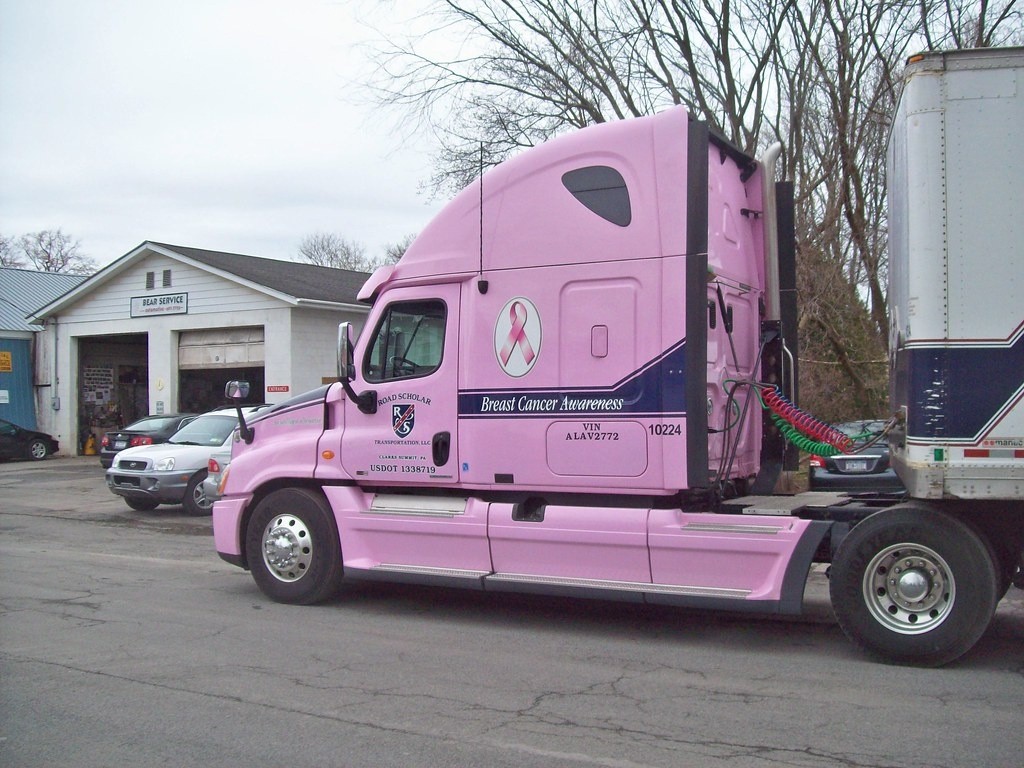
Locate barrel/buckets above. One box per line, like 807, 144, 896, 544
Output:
84, 434, 97, 455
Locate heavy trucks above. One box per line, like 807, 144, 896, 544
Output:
213, 49, 1024, 671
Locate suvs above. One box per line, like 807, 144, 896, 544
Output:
106, 404, 276, 517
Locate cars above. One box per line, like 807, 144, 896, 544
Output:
100, 413, 202, 471
808, 417, 910, 503
0, 419, 59, 465
